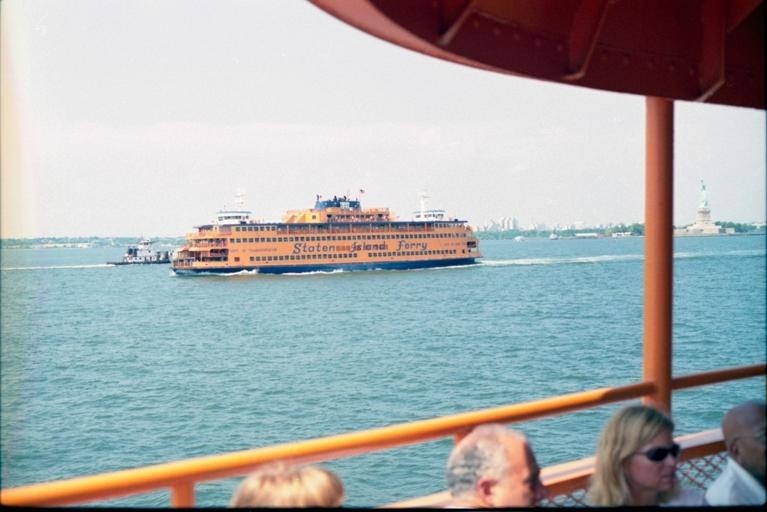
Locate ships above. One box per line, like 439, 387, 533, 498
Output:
169, 189, 485, 276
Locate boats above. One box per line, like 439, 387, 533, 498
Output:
549, 233, 559, 240
513, 235, 524, 241
104, 238, 171, 266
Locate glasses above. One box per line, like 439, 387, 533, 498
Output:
635, 445, 679, 460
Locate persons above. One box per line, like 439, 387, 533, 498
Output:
586, 409, 708, 505
705, 398, 765, 506
441, 422, 546, 509
227, 461, 345, 509
